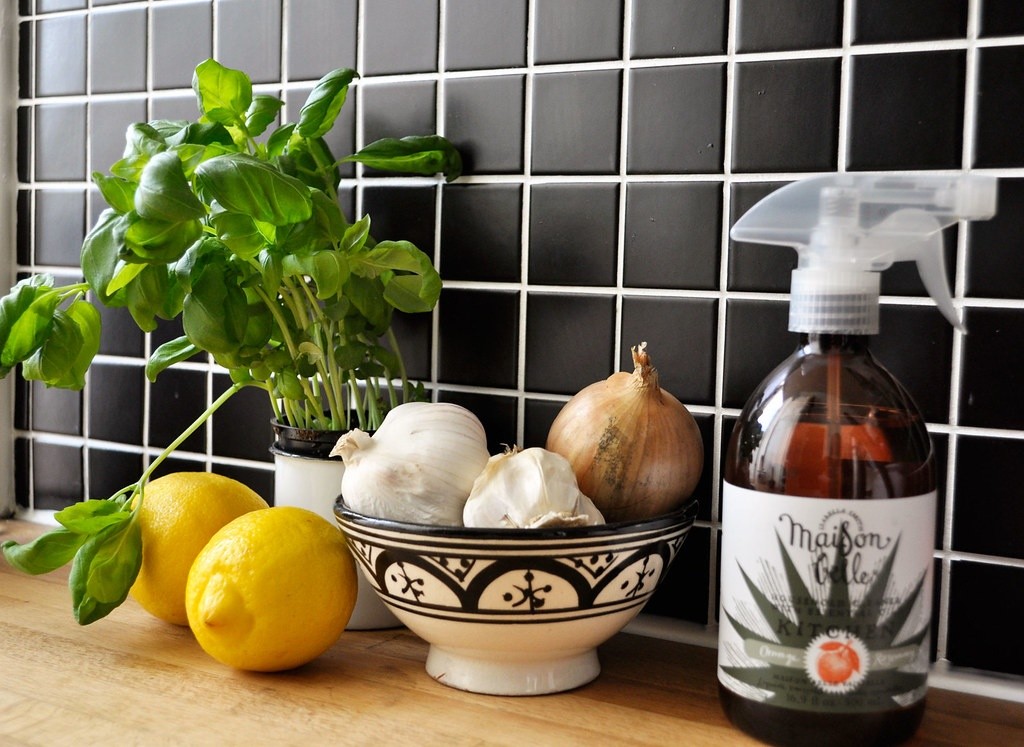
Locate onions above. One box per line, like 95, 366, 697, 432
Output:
546, 342, 704, 522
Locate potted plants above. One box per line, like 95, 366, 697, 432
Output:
0, 53, 460, 641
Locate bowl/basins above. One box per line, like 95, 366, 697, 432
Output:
334, 490, 699, 695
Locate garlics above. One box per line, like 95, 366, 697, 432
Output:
329, 401, 604, 532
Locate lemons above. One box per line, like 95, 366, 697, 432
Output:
122, 472, 359, 672
